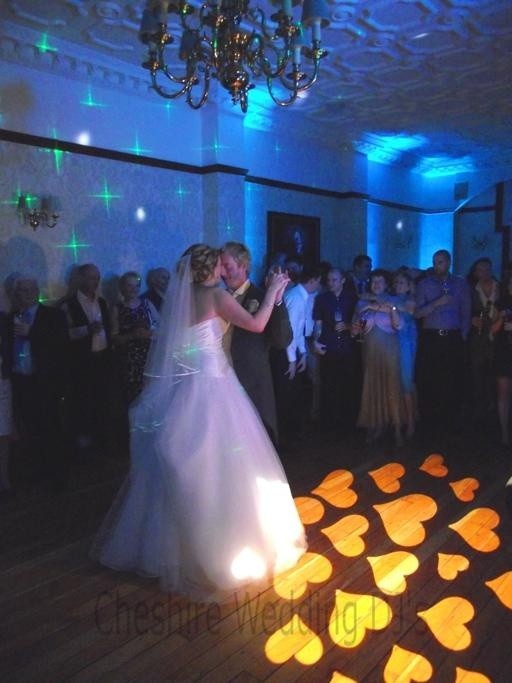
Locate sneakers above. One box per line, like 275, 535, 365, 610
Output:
360, 416, 417, 449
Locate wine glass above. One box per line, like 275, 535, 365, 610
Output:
334, 311, 345, 339
441, 280, 449, 305
356, 316, 369, 342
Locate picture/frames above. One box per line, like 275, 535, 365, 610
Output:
264, 208, 323, 270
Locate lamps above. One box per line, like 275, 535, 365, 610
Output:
134, 0, 334, 117
15, 190, 66, 231
468, 232, 490, 251
